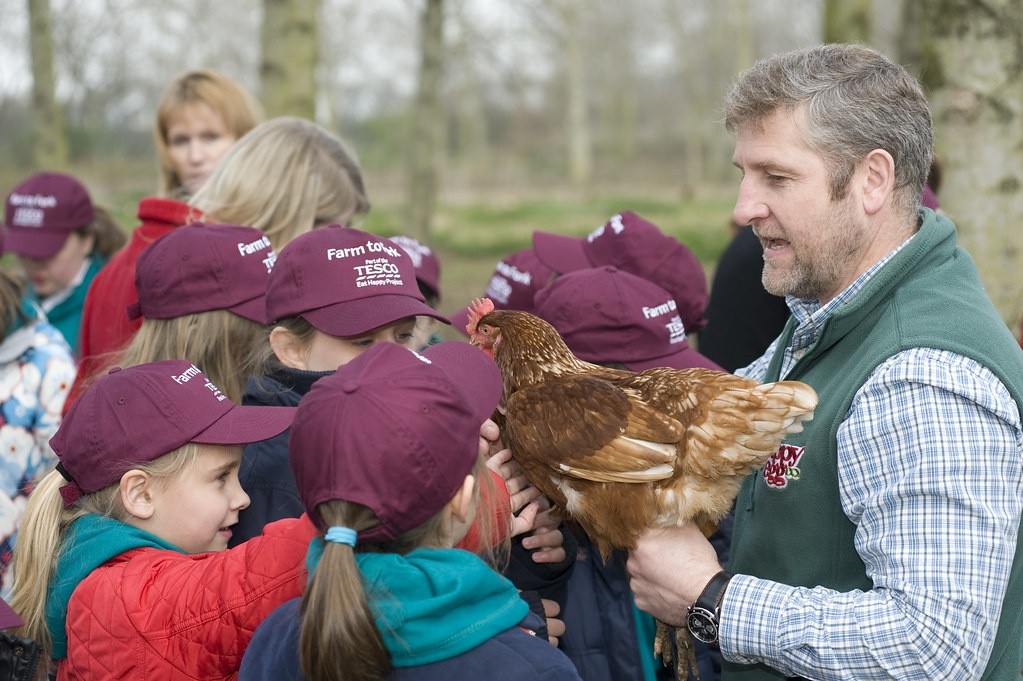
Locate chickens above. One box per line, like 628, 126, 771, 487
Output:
466, 297, 819, 681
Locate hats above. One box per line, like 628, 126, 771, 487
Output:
0, 172, 96, 256
381, 234, 442, 301
266, 223, 451, 337
289, 340, 504, 548
532, 266, 730, 375
449, 250, 565, 341
49, 360, 298, 506
127, 220, 280, 325
922, 183, 939, 212
533, 210, 709, 336
0, 598, 24, 629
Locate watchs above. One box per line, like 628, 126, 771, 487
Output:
684, 570, 731, 646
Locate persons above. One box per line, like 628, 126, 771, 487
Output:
0, 68, 728, 680
624, 42, 1023, 681
236, 339, 583, 681
9, 359, 542, 681
